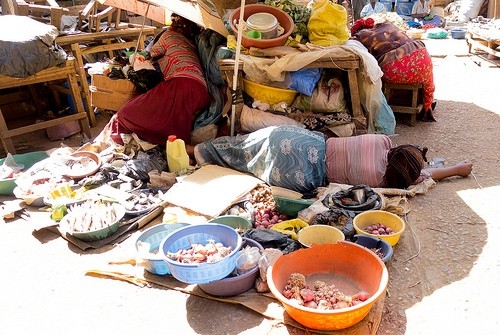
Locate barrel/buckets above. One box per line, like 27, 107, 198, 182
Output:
246, 13, 278, 39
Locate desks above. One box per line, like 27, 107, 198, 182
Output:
466, 32, 500, 68
55, 28, 154, 125
218, 52, 360, 128
1, 58, 92, 156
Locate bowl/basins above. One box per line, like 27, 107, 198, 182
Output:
207, 215, 253, 237
451, 29, 466, 39
134, 222, 191, 275
325, 189, 381, 213
43, 184, 98, 207
0, 151, 48, 195
274, 197, 314, 215
65, 152, 100, 179
229, 3, 294, 48
353, 210, 406, 247
346, 234, 393, 261
267, 240, 389, 331
160, 223, 241, 284
243, 78, 299, 106
198, 237, 264, 296
25, 178, 49, 206
59, 202, 126, 242
297, 223, 345, 248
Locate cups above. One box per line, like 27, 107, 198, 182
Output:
246, 30, 261, 40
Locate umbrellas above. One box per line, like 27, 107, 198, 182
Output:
129, 0, 229, 69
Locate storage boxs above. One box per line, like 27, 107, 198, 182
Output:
90, 74, 135, 111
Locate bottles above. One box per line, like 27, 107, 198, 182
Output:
166, 135, 190, 173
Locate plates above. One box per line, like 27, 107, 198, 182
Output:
121, 188, 164, 215
270, 186, 303, 200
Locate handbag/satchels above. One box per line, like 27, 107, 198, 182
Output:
290, 69, 347, 113
306, 0, 351, 47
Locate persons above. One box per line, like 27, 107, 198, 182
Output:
186, 125, 472, 195
360, 0, 386, 18
411, 0, 431, 19
93, 12, 208, 155
351, 18, 435, 121
342, 0, 351, 19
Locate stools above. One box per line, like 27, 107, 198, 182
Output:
384, 83, 424, 125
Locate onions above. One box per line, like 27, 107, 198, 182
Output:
364, 222, 396, 236
247, 185, 288, 229
284, 286, 369, 311
167, 240, 234, 265
